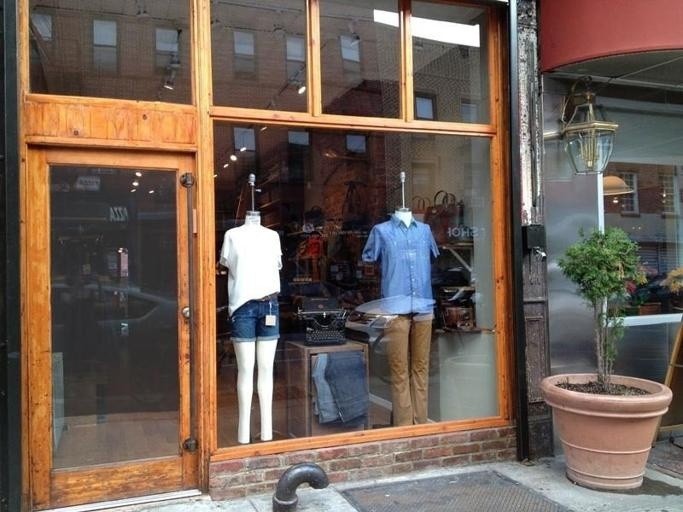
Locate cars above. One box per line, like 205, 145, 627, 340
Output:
286, 277, 345, 343
346, 280, 472, 345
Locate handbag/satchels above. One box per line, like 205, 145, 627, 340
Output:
304, 205, 323, 228
411, 189, 463, 244
299, 308, 350, 345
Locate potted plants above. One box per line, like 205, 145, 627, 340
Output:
540, 224, 671, 490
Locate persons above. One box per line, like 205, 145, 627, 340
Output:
362, 208, 441, 427
220, 212, 282, 444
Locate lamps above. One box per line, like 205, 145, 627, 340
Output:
557, 75, 618, 177
